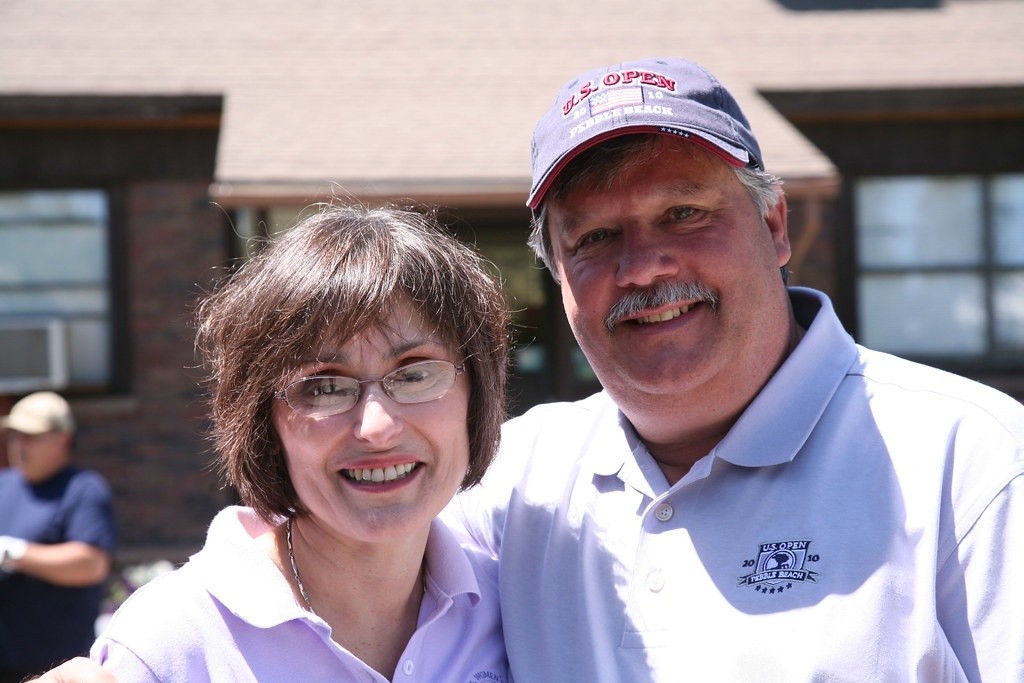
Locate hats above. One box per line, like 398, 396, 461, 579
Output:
525, 58, 766, 263
0, 390, 76, 436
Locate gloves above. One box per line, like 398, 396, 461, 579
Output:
0, 535, 28, 571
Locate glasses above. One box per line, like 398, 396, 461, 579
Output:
272, 360, 468, 418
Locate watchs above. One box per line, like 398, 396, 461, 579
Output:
0, 537, 27, 573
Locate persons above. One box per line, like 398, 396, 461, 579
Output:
0, 52, 510, 683
443, 58, 1024, 683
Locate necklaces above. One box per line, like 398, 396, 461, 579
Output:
286, 519, 427, 616
